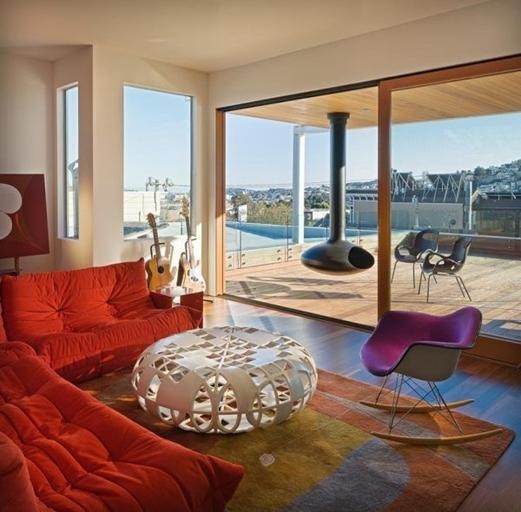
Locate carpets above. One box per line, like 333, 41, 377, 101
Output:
70, 365, 516, 511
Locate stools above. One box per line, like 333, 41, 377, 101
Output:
133, 323, 317, 429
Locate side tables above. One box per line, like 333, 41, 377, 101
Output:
150, 286, 203, 329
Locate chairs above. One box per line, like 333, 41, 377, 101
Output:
391, 228, 473, 306
356, 306, 502, 443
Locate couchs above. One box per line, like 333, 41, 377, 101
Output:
2, 257, 204, 380
1, 328, 244, 510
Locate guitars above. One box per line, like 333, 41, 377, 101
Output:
177, 197, 206, 294
146, 214, 175, 290
